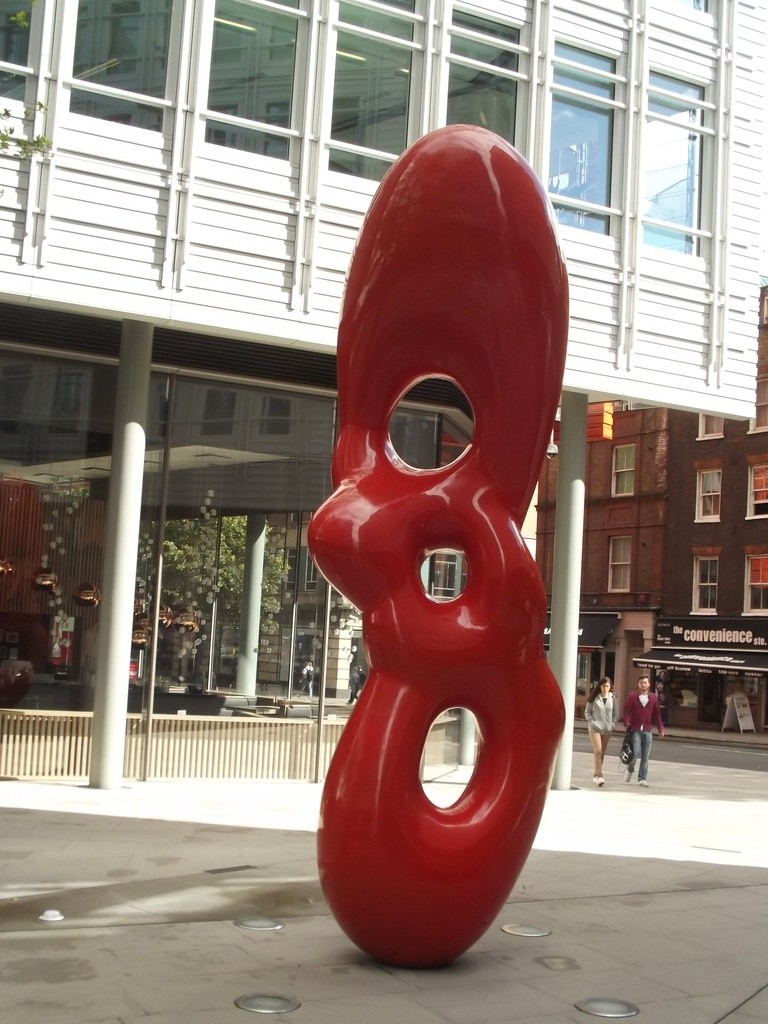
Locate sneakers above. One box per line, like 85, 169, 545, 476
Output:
624, 768, 632, 782
637, 779, 650, 787
592, 775, 605, 786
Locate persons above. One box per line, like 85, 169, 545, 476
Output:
584, 676, 619, 787
356, 666, 368, 695
623, 676, 665, 787
296, 661, 313, 699
346, 667, 360, 704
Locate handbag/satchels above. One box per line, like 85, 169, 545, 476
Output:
619, 728, 634, 765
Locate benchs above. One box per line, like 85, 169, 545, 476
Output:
164, 688, 349, 716
